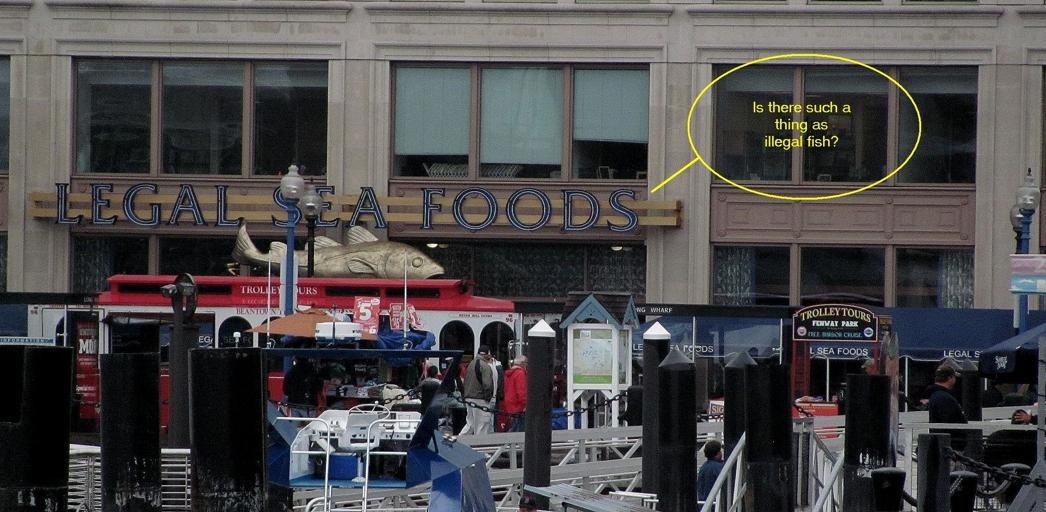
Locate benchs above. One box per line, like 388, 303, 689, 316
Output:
523, 482, 656, 512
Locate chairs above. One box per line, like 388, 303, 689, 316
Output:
337, 413, 380, 483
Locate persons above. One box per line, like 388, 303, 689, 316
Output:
697, 440, 724, 500
987, 408, 1037, 449
929, 365, 969, 451
283, 344, 528, 435
860, 358, 879, 374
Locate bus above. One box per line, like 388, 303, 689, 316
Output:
94, 274, 516, 430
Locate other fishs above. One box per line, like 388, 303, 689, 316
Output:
230, 224, 446, 280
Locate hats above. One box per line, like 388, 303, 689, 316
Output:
478, 345, 490, 355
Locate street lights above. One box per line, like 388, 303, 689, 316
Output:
1016, 167, 1042, 338
1009, 200, 1028, 337
279, 165, 303, 374
300, 176, 324, 277
